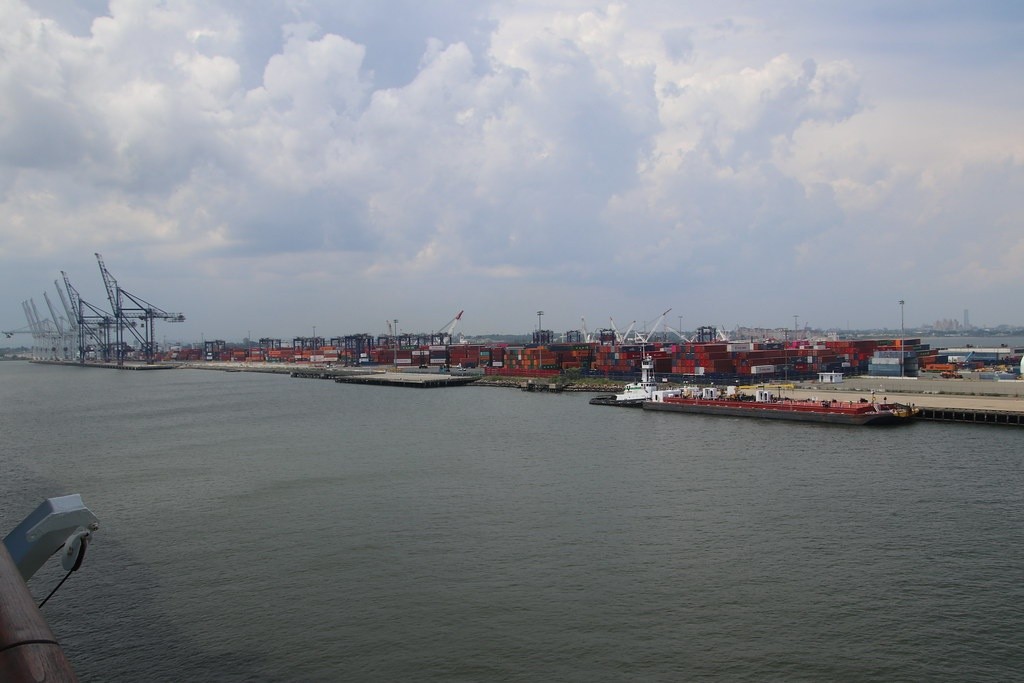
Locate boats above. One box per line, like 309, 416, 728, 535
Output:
640, 384, 921, 426
613, 353, 662, 403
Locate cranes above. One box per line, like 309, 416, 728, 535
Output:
431, 309, 464, 345
578, 307, 697, 345
0, 252, 186, 366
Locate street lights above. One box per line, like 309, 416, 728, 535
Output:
394, 319, 398, 373
313, 325, 317, 361
678, 316, 682, 345
201, 332, 204, 359
537, 310, 544, 368
248, 329, 251, 362
899, 300, 905, 376
793, 315, 800, 340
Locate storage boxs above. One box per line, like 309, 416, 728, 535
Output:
161, 339, 1024, 403
